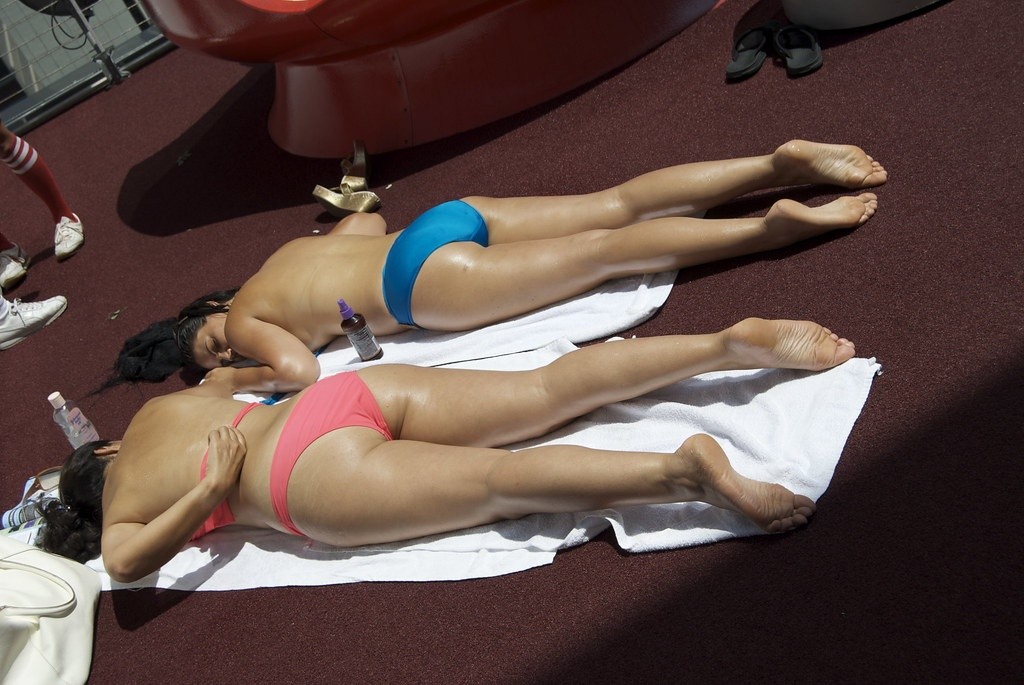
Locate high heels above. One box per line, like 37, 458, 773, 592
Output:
312, 185, 381, 218
340, 138, 368, 191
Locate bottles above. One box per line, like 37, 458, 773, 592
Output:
48, 392, 101, 449
337, 298, 383, 361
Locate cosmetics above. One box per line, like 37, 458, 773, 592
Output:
47, 391, 100, 450
337, 298, 384, 362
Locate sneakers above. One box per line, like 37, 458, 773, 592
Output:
54, 212, 84, 259
0, 241, 31, 288
0, 295, 67, 350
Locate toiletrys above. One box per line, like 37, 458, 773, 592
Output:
48, 391, 100, 449
336, 299, 384, 362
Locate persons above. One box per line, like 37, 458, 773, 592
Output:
0, 286, 67, 349
106, 135, 894, 403
0, 126, 86, 292
39, 311, 857, 586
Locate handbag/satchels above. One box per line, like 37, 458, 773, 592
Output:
0, 533, 103, 685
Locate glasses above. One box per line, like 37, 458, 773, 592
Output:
22, 466, 63, 505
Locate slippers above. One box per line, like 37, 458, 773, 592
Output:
774, 24, 823, 76
725, 23, 771, 78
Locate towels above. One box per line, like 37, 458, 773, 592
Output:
82, 203, 883, 593
